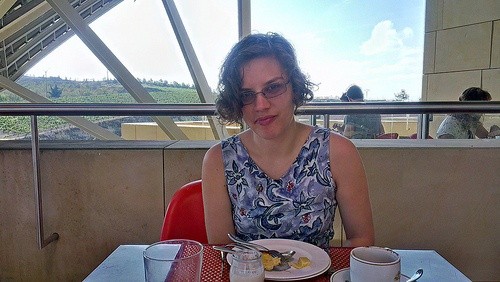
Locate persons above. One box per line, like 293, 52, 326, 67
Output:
436, 87, 500, 139
202, 32, 375, 247
333, 85, 382, 139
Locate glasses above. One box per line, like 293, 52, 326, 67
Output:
238, 81, 289, 104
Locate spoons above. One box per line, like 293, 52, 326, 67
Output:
213, 246, 295, 260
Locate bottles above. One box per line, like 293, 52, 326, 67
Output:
230, 251, 264, 282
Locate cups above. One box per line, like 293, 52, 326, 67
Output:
350, 247, 401, 282
143, 239, 204, 282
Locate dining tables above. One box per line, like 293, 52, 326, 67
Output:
82, 244, 473, 281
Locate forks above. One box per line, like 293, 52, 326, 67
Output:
227, 243, 290, 271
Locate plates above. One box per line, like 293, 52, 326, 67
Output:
330, 267, 417, 282
227, 239, 331, 281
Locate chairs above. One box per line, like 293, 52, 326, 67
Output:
159, 179, 208, 246
